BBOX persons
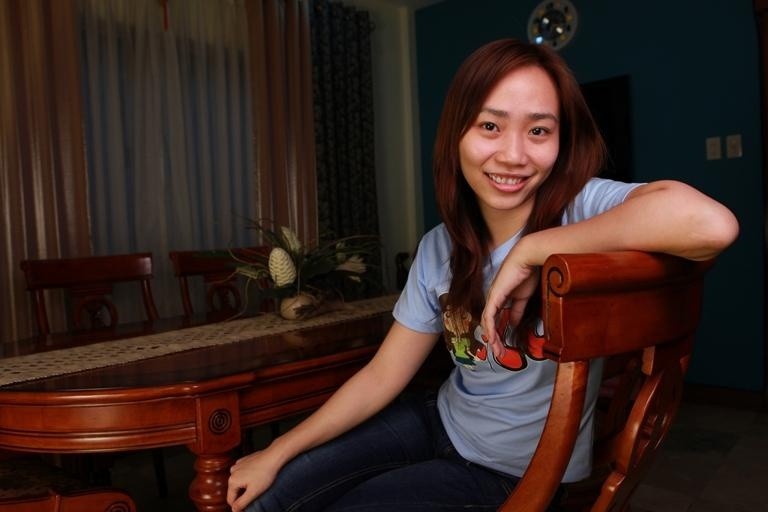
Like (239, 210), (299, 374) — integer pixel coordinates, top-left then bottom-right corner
(226, 39), (741, 511)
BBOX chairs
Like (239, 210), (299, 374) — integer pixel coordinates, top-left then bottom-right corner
(168, 249), (294, 454)
(17, 254), (195, 510)
(499, 250), (706, 511)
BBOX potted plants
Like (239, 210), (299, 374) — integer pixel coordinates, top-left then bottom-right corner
(207, 225), (383, 322)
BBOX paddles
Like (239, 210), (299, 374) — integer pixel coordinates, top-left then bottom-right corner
(628, 426), (741, 512)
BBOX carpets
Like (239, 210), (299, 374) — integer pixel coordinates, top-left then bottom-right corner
(628, 426), (741, 512)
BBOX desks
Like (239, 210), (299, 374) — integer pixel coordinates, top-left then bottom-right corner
(2, 286), (452, 510)
